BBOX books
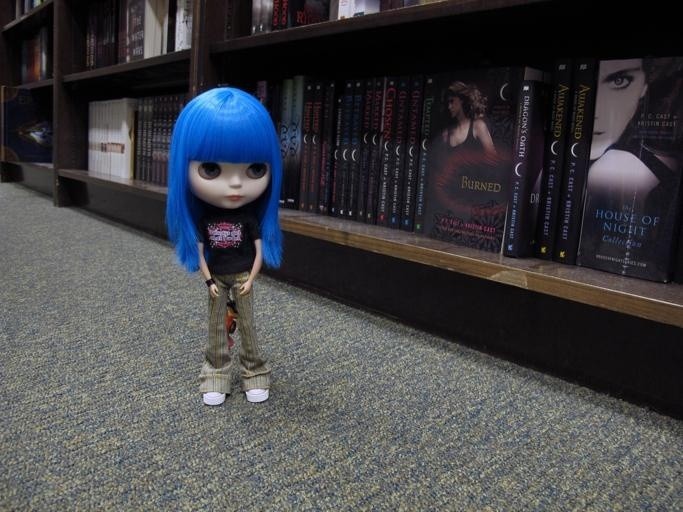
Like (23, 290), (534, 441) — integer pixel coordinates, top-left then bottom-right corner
(3, 1), (680, 284)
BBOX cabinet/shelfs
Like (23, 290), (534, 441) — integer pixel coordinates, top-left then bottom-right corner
(0, 0), (683, 416)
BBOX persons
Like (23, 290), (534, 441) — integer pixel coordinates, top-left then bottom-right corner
(163, 86), (286, 408)
